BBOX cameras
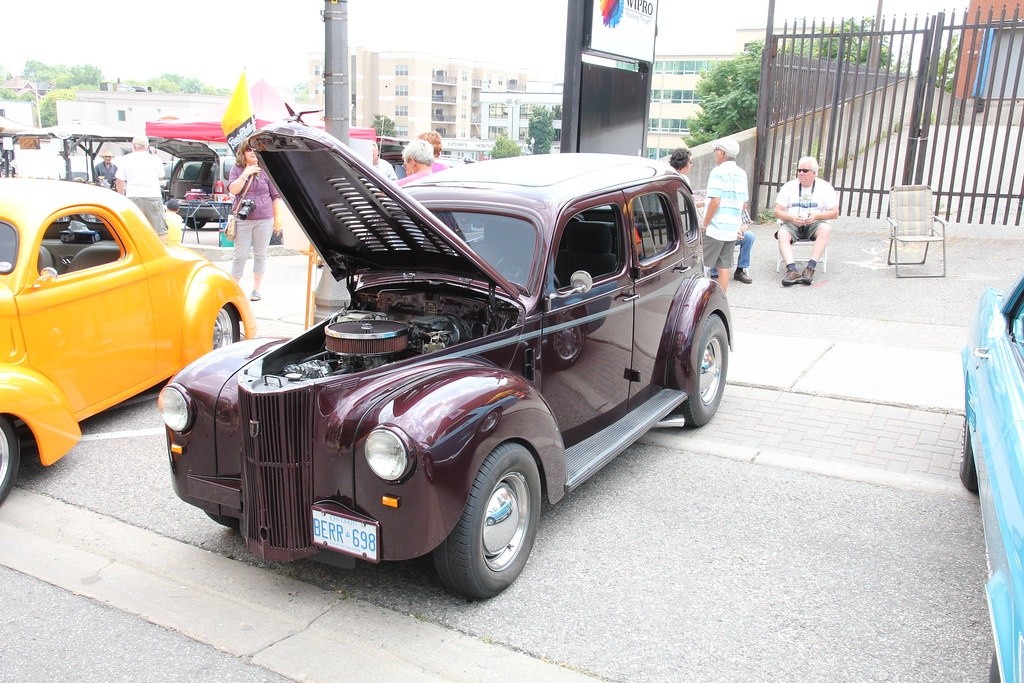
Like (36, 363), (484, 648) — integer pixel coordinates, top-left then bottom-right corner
(798, 224), (809, 234)
(238, 199), (256, 220)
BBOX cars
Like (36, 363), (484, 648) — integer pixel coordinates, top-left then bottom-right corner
(155, 119), (738, 602)
(959, 270), (1024, 683)
(0, 178), (256, 505)
(146, 136), (244, 231)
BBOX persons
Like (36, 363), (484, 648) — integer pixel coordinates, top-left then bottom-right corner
(774, 156), (840, 285)
(226, 138), (281, 301)
(95, 151), (117, 188)
(417, 131), (448, 174)
(695, 137), (749, 293)
(669, 147), (692, 184)
(163, 200), (183, 246)
(391, 140), (433, 187)
(371, 142), (398, 181)
(114, 135), (165, 237)
(710, 202), (755, 284)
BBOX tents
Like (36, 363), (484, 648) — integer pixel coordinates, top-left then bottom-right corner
(146, 116), (377, 143)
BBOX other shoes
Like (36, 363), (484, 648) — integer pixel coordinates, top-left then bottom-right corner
(782, 270), (804, 286)
(801, 266), (815, 285)
(251, 290), (261, 301)
(734, 271), (752, 283)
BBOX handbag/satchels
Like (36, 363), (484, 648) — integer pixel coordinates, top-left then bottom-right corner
(224, 214), (237, 241)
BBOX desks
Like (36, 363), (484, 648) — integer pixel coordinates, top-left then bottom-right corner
(181, 201), (234, 247)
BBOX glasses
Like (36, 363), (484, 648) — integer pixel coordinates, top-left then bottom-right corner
(797, 169), (814, 173)
(246, 147), (253, 152)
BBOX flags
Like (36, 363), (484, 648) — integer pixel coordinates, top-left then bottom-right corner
(220, 72), (255, 158)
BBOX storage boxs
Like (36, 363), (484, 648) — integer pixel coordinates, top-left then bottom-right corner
(220, 232), (234, 247)
(186, 191), (206, 203)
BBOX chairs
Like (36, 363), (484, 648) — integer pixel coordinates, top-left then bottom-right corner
(483, 221), (530, 255)
(0, 242), (53, 275)
(694, 190), (748, 276)
(554, 221), (616, 289)
(887, 185), (947, 278)
(777, 219), (828, 272)
(68, 241), (121, 272)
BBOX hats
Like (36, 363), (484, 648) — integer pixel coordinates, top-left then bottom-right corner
(166, 199), (179, 211)
(712, 138), (740, 156)
(99, 150), (115, 157)
